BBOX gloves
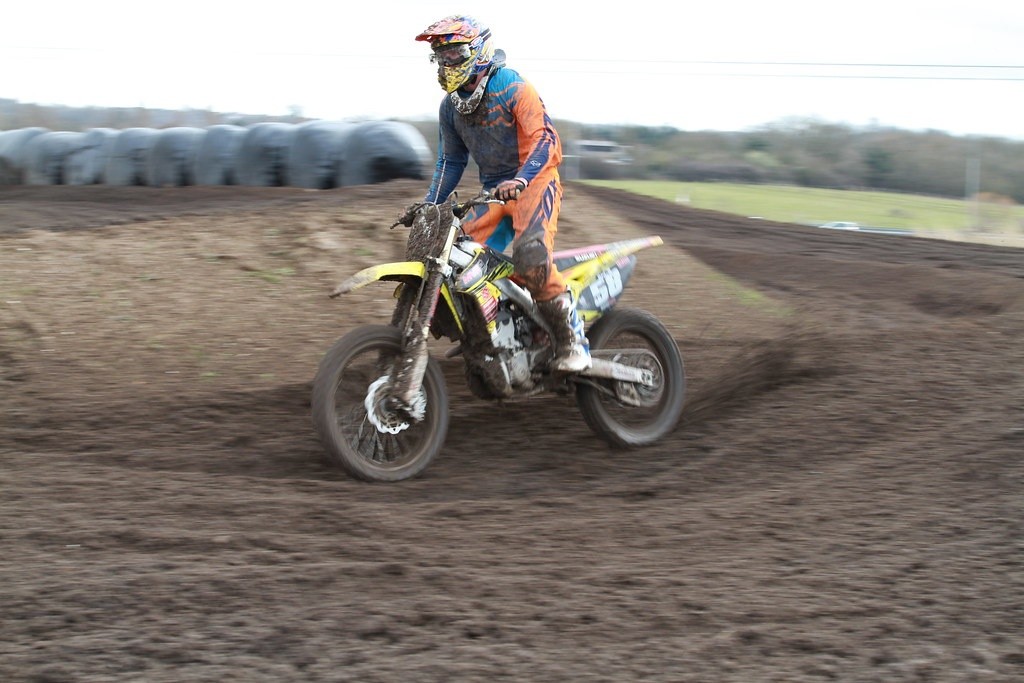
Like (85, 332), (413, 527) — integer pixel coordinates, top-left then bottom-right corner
(493, 177), (529, 202)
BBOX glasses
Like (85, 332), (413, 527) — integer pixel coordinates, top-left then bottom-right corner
(427, 45), (471, 65)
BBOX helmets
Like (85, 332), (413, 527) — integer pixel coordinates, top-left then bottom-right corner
(414, 14), (496, 95)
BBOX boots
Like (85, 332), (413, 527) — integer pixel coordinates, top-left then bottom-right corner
(535, 284), (593, 372)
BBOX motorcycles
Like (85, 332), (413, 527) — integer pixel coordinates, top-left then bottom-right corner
(312, 188), (686, 482)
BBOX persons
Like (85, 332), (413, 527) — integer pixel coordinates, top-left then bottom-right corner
(398, 15), (592, 371)
